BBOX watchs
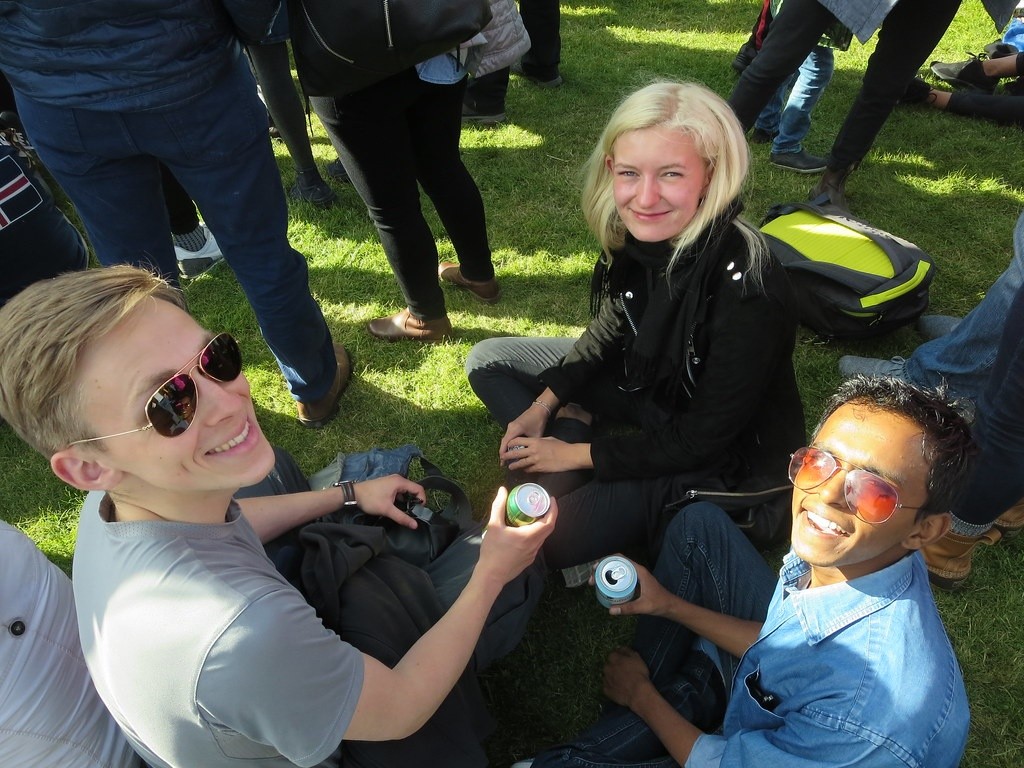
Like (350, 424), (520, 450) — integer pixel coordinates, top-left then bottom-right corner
(334, 480), (357, 508)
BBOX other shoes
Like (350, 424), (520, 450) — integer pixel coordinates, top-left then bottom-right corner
(732, 44), (757, 72)
(285, 178), (335, 207)
(512, 62), (562, 87)
(461, 104), (506, 123)
(330, 159), (349, 181)
(551, 561), (597, 588)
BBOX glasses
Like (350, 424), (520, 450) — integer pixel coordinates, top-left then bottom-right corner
(788, 447), (930, 525)
(68, 332), (243, 448)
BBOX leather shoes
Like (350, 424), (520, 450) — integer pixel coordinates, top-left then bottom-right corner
(769, 144), (828, 172)
(366, 307), (453, 342)
(750, 126), (779, 143)
(437, 259), (502, 304)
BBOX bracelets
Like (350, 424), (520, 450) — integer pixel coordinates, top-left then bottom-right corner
(532, 400), (554, 417)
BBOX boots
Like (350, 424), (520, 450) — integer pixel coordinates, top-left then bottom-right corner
(915, 520), (1002, 591)
(997, 498), (1024, 542)
(810, 156), (858, 213)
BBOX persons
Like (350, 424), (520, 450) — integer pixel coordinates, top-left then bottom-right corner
(837, 205), (1024, 588)
(465, 81), (806, 570)
(726, 0), (1023, 219)
(512, 372), (971, 768)
(0, 519), (141, 768)
(896, 52), (1024, 123)
(0, 0), (353, 426)
(461, 0), (562, 124)
(244, 0), (502, 343)
(0, 264), (558, 768)
(0, 144), (89, 305)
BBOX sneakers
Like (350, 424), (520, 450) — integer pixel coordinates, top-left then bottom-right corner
(930, 52), (1000, 93)
(296, 344), (352, 428)
(838, 354), (908, 384)
(919, 314), (963, 339)
(173, 224), (224, 280)
(899, 75), (937, 105)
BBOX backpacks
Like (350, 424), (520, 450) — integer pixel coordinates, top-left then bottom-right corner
(753, 193), (934, 341)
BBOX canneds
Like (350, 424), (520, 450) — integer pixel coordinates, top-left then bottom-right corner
(481, 482), (550, 539)
(506, 444), (527, 472)
(594, 555), (637, 609)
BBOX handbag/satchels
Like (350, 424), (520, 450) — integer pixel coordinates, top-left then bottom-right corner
(285, 0), (493, 98)
(308, 444), (419, 524)
